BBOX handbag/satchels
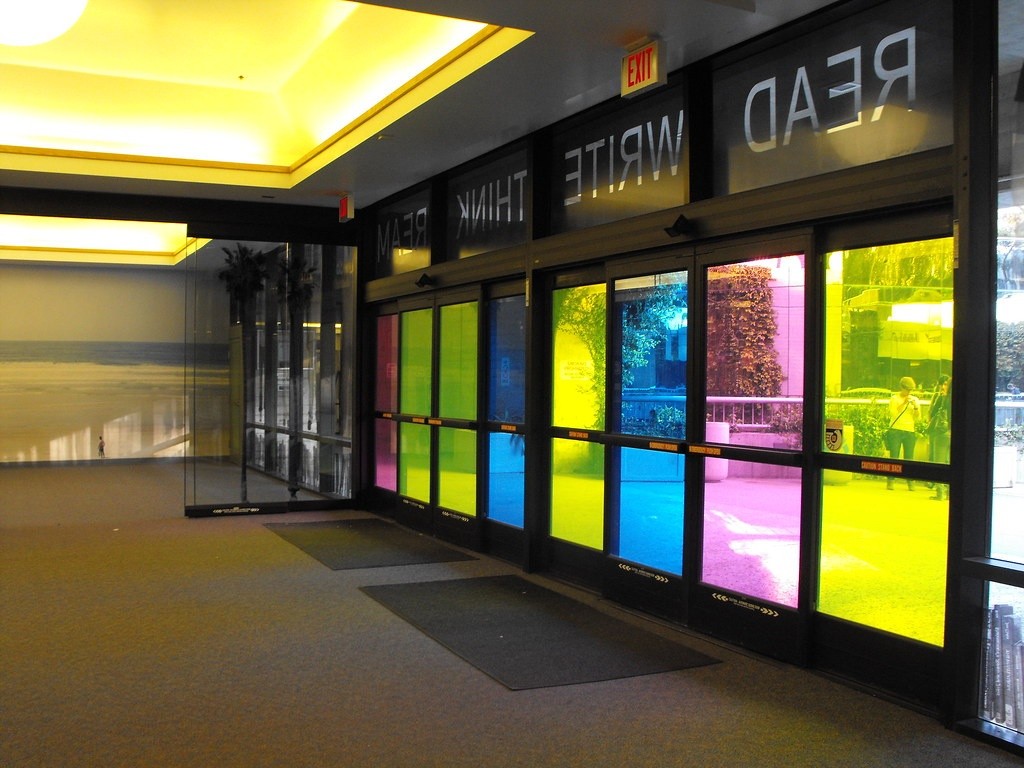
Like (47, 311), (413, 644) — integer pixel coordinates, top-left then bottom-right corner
(883, 432), (890, 450)
(923, 408), (948, 436)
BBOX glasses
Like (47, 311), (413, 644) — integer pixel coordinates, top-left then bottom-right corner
(902, 386), (915, 392)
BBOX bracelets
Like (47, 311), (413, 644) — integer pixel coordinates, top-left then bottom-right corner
(914, 407), (919, 410)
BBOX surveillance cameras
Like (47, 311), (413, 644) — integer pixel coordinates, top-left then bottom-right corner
(415, 273), (430, 287)
(664, 215), (688, 239)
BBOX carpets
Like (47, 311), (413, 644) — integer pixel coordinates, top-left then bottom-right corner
(357, 574), (726, 690)
(263, 518), (480, 572)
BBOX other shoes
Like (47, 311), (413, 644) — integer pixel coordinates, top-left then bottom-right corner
(908, 480), (916, 491)
(887, 479), (894, 490)
(930, 494), (944, 501)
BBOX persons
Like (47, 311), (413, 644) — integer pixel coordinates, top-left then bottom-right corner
(1007, 383), (1020, 399)
(926, 375), (952, 500)
(886, 376), (920, 491)
(98, 436), (105, 458)
